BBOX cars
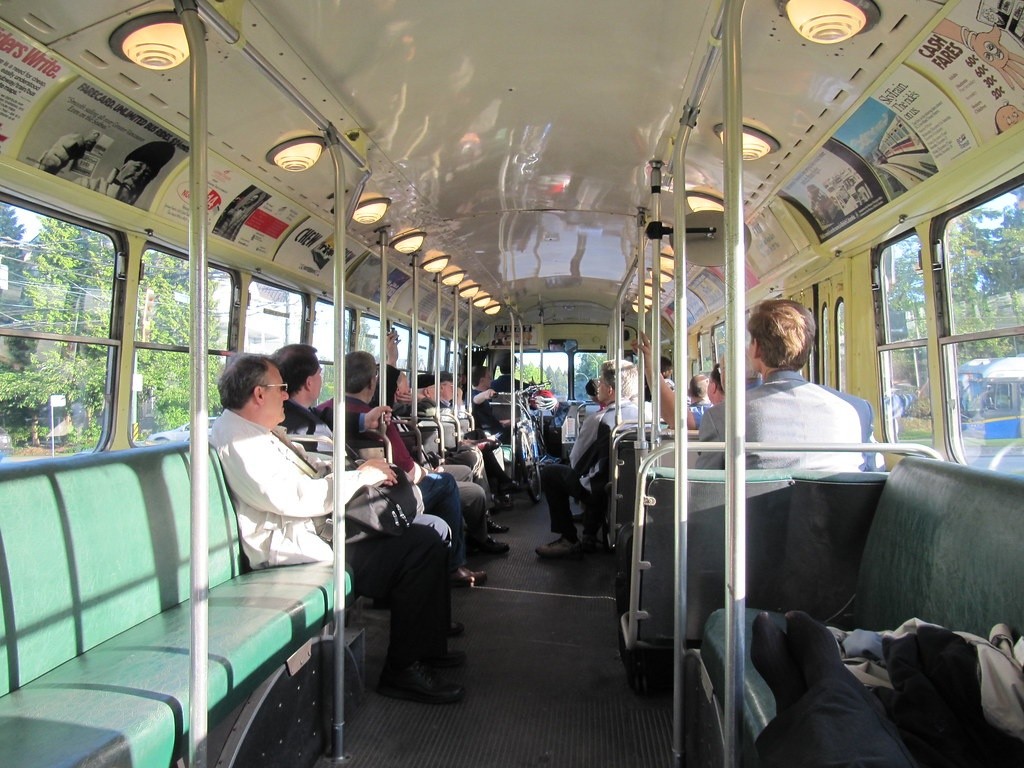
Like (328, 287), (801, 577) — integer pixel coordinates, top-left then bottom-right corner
(147, 416), (218, 445)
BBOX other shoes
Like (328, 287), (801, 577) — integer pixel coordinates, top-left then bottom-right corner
(508, 480), (520, 489)
(499, 494), (513, 511)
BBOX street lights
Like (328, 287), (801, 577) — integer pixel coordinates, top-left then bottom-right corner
(577, 353), (598, 378)
(575, 373), (590, 401)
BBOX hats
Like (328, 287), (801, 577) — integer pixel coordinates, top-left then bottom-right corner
(418, 374), (443, 388)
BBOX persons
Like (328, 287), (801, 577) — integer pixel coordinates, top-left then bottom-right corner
(534, 337), (709, 558)
(491, 354), (530, 392)
(639, 331), (762, 431)
(694, 300), (886, 471)
(272, 344), (465, 639)
(368, 331), (528, 554)
(218, 195), (260, 241)
(84, 132), (100, 152)
(749, 612), (919, 768)
(318, 351), (486, 588)
(209, 355), (469, 702)
(37, 133), (175, 205)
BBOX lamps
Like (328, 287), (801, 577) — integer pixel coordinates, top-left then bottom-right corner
(419, 256), (451, 273)
(482, 304), (502, 315)
(632, 303), (650, 314)
(442, 271), (467, 286)
(686, 191), (725, 213)
(713, 124), (780, 161)
(108, 11), (207, 70)
(460, 284), (480, 297)
(390, 232), (428, 254)
(644, 283), (666, 298)
(352, 198), (392, 225)
(265, 136), (326, 173)
(776, 0), (881, 45)
(646, 268), (673, 282)
(635, 294), (652, 306)
(660, 253), (674, 269)
(473, 295), (493, 307)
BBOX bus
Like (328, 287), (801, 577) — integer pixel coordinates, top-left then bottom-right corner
(957, 356), (1024, 441)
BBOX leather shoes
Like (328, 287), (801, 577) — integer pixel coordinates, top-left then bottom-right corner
(487, 518), (510, 533)
(479, 538), (510, 555)
(448, 566), (486, 588)
(450, 621), (464, 637)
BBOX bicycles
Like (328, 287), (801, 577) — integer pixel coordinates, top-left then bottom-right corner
(491, 382), (551, 505)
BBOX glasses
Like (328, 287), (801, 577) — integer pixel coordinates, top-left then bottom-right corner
(262, 382), (288, 392)
(484, 376), (492, 380)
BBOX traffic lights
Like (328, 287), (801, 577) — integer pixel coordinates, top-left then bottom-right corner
(142, 321), (153, 351)
(142, 289), (162, 325)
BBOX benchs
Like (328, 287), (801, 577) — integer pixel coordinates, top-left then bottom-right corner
(608, 420), (1024, 740)
(0, 407), (518, 768)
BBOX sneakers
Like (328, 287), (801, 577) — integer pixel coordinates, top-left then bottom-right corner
(434, 652), (466, 669)
(535, 537), (580, 558)
(581, 536), (594, 552)
(376, 665), (463, 705)
